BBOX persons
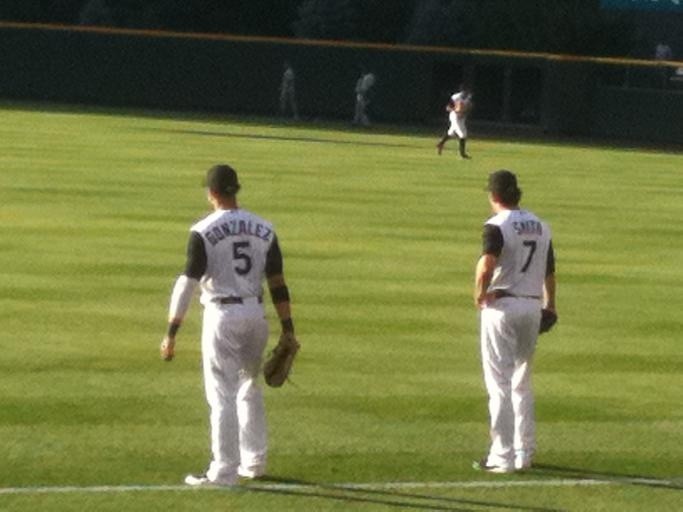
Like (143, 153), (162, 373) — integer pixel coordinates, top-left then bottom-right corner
(437, 81), (474, 158)
(160, 165), (295, 485)
(351, 60), (376, 127)
(279, 60), (301, 122)
(471, 170), (558, 474)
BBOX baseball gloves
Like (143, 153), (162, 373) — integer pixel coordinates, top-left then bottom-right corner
(539, 309), (557, 335)
(263, 331), (300, 388)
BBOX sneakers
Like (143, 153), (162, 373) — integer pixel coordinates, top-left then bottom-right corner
(472, 456), (513, 474)
(236, 463), (266, 478)
(461, 153), (472, 159)
(437, 142), (443, 155)
(515, 453), (532, 471)
(185, 469), (239, 487)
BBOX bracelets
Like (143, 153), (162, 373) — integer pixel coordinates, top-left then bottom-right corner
(165, 322), (180, 337)
(281, 318), (294, 332)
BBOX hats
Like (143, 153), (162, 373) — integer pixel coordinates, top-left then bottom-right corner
(482, 168), (517, 194)
(200, 164), (239, 191)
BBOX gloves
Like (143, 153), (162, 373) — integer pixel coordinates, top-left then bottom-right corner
(159, 333), (174, 361)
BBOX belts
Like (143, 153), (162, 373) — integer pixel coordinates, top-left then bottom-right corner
(494, 290), (539, 299)
(223, 297), (262, 306)
